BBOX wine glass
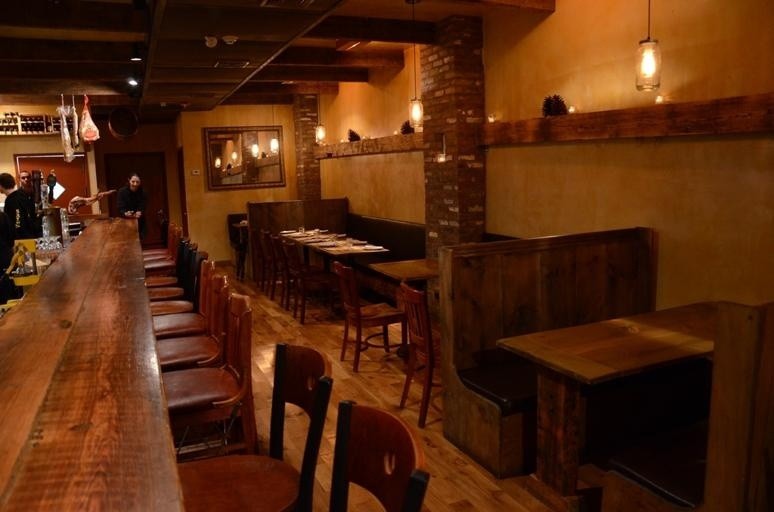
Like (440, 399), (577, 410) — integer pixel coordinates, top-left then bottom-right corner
(34, 236), (64, 251)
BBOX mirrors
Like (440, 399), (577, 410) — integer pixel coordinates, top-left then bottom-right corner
(202, 126), (286, 191)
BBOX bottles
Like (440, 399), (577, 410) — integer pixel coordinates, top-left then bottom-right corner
(0, 112), (77, 131)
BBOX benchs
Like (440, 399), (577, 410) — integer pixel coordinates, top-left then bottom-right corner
(328, 212), (519, 306)
(227, 197), (350, 282)
(438, 225), (657, 480)
(601, 300), (774, 512)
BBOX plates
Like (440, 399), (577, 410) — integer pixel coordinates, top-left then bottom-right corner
(280, 227), (384, 252)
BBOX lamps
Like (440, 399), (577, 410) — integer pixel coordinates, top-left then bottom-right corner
(409, 43), (424, 128)
(636, 0), (662, 91)
(213, 130), (279, 168)
(315, 92), (326, 144)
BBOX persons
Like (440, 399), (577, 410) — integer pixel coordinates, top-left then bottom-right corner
(117, 171), (148, 240)
(18, 171), (34, 199)
(0, 172), (35, 239)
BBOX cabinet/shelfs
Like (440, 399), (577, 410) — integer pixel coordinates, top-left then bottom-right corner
(0, 113), (79, 136)
